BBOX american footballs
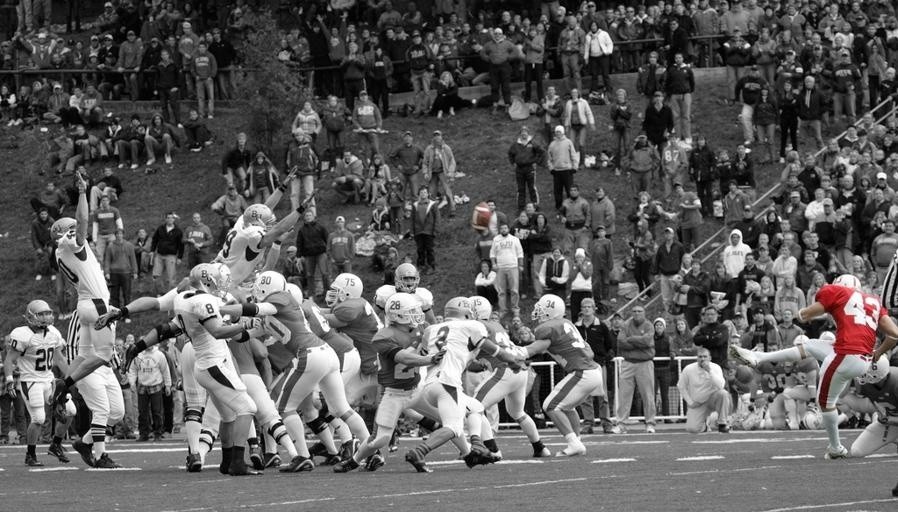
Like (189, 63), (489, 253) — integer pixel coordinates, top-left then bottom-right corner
(472, 200), (491, 234)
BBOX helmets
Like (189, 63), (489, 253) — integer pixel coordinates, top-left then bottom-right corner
(468, 296), (492, 321)
(249, 271), (287, 303)
(190, 263), (232, 294)
(243, 204), (276, 237)
(803, 406), (822, 431)
(25, 299), (55, 330)
(51, 218), (77, 241)
(385, 292), (425, 324)
(332, 273), (362, 300)
(793, 334), (809, 346)
(819, 331), (836, 340)
(531, 294), (564, 322)
(394, 264), (420, 293)
(443, 296), (477, 321)
(287, 283), (303, 306)
(832, 274), (861, 289)
(856, 350), (890, 386)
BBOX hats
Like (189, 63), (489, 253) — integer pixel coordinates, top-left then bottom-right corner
(754, 307), (764, 314)
(56, 30), (134, 43)
(733, 311), (743, 317)
(653, 317), (667, 329)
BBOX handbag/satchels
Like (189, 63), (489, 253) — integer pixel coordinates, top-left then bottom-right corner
(672, 290), (688, 306)
(668, 303), (683, 315)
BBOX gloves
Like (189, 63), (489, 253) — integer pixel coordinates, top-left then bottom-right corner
(6, 376), (16, 398)
(430, 351), (446, 363)
(76, 171), (87, 193)
(279, 165), (297, 193)
(296, 191), (319, 215)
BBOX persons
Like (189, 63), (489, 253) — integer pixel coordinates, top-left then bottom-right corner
(0, 0), (897, 474)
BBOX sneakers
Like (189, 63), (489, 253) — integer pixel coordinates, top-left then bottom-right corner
(47, 378), (69, 424)
(718, 424), (729, 432)
(824, 446), (848, 460)
(93, 308), (123, 330)
(1, 427), (180, 468)
(729, 344), (759, 368)
(7, 115), (215, 176)
(186, 438), (502, 475)
(581, 421), (656, 434)
(121, 344), (138, 374)
(532, 447), (551, 458)
(556, 447), (585, 456)
